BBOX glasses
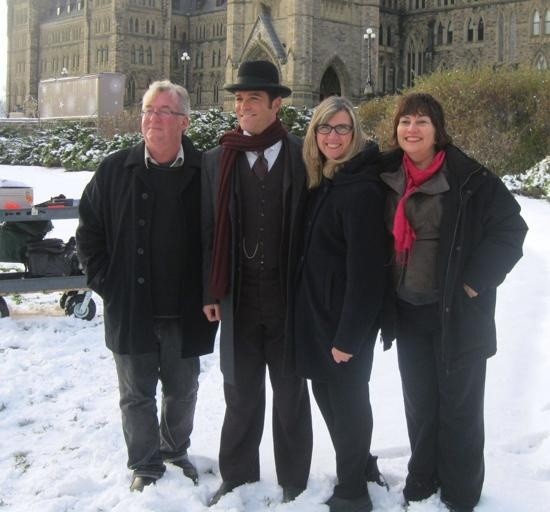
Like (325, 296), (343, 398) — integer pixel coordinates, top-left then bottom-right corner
(140, 108), (185, 120)
(316, 123), (354, 135)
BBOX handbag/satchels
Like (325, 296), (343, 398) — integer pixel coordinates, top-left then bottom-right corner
(19, 235), (85, 277)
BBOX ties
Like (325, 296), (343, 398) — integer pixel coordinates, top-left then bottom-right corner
(250, 148), (269, 182)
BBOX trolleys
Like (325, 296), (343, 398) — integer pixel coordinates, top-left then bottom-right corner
(0, 198), (98, 320)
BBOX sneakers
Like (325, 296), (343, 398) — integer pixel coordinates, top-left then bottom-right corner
(173, 460), (198, 485)
(207, 481), (244, 505)
(281, 486), (307, 504)
(325, 495), (375, 511)
(130, 476), (155, 492)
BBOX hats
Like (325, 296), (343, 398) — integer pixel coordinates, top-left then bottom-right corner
(223, 61), (293, 99)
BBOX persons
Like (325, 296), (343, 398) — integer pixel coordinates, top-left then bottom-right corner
(200, 59), (315, 508)
(294, 96), (388, 511)
(76, 80), (218, 491)
(379, 93), (528, 512)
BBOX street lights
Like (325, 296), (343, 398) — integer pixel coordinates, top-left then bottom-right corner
(60, 67), (69, 77)
(181, 51), (191, 87)
(363, 27), (375, 100)
